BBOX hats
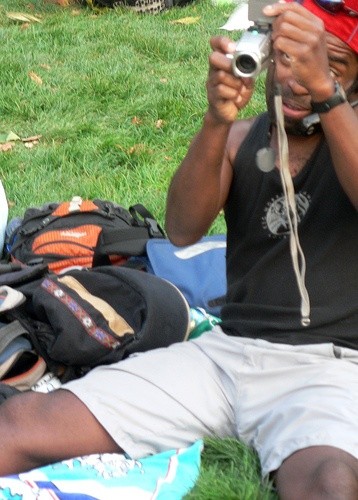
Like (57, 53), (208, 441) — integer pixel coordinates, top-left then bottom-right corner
(284, 0), (358, 54)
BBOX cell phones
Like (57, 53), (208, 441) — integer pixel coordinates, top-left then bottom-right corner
(300, 99), (358, 137)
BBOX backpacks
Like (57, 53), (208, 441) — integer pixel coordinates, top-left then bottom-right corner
(0, 311), (46, 404)
(4, 195), (166, 275)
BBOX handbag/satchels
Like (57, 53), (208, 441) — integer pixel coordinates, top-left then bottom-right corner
(145, 233), (228, 317)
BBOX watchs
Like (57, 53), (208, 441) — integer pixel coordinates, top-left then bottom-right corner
(310, 80), (348, 113)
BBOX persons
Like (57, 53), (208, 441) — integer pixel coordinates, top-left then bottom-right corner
(1, 0), (358, 496)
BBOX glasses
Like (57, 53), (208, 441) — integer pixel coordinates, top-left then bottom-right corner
(313, 0), (358, 20)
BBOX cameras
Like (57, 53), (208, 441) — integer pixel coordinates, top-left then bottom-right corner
(223, 0), (280, 77)
